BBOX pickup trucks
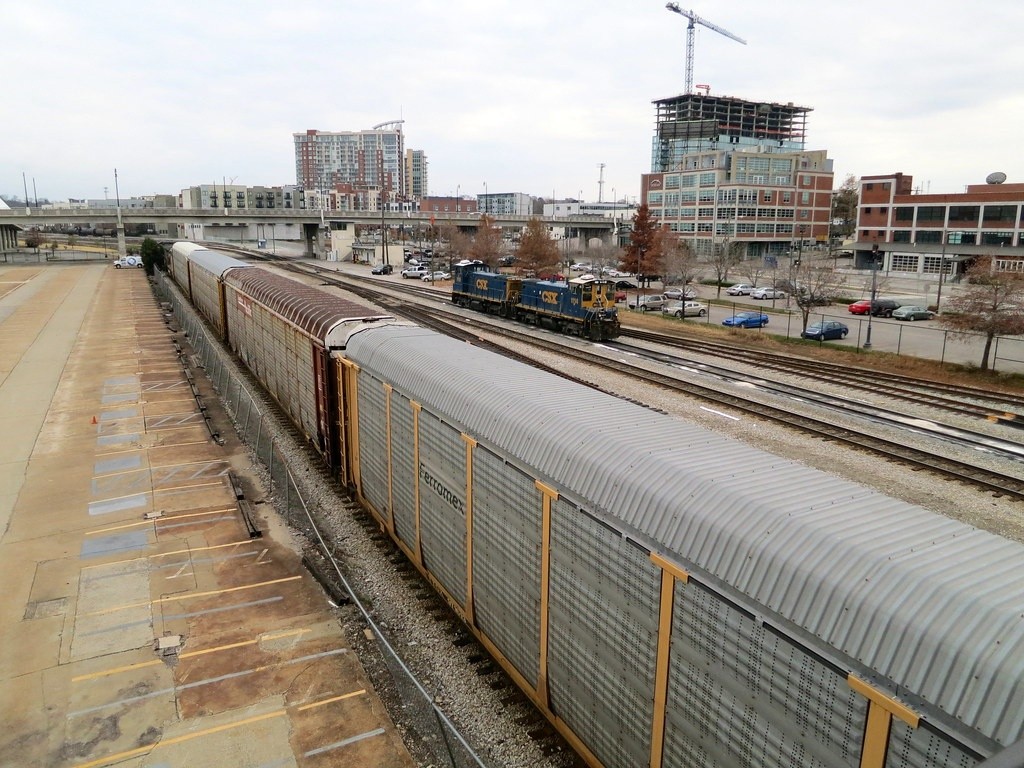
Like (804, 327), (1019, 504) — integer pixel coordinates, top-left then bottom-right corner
(402, 266), (430, 279)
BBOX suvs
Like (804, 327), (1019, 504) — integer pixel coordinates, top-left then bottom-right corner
(629, 295), (669, 311)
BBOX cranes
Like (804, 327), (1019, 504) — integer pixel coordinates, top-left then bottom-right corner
(665, 2), (747, 96)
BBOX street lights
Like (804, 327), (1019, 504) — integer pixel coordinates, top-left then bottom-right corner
(455, 177), (642, 220)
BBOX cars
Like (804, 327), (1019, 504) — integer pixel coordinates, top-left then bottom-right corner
(664, 286), (696, 301)
(750, 287), (786, 300)
(372, 264), (393, 275)
(892, 306), (934, 322)
(113, 257), (144, 269)
(422, 271), (451, 282)
(849, 300), (871, 316)
(403, 245), (483, 270)
(801, 320), (849, 342)
(615, 280), (638, 303)
(662, 300), (708, 319)
(871, 298), (902, 318)
(495, 254), (662, 282)
(726, 283), (754, 296)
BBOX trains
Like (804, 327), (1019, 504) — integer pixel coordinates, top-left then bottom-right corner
(167, 239), (1024, 768)
(450, 259), (621, 343)
